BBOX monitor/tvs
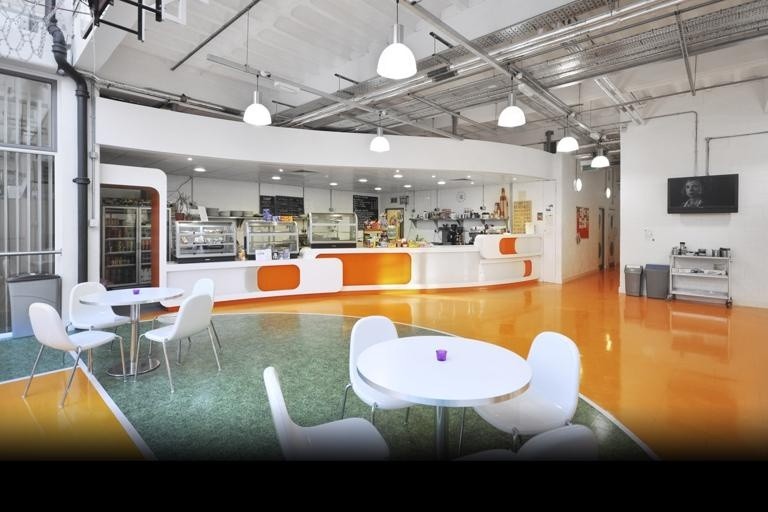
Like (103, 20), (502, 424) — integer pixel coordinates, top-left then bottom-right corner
(667, 174), (738, 214)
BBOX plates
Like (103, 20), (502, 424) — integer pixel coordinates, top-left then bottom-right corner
(207, 208), (254, 217)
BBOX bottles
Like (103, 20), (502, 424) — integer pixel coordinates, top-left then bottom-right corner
(106, 219), (150, 265)
(273, 248), (290, 260)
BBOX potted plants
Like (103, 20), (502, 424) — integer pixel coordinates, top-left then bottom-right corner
(175, 189), (193, 221)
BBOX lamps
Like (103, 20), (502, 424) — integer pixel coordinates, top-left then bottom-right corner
(370, 114), (390, 154)
(376, 0), (417, 81)
(497, 76), (527, 129)
(243, 76), (271, 126)
(556, 116), (579, 152)
(590, 142), (610, 168)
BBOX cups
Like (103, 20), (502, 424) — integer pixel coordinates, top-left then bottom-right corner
(672, 242), (731, 257)
(436, 349), (447, 362)
(131, 287), (140, 295)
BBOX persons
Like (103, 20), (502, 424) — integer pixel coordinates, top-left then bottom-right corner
(681, 178), (708, 209)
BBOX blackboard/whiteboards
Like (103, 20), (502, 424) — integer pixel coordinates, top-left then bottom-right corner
(353, 195), (378, 230)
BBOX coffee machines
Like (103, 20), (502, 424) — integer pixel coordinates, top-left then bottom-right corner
(448, 224), (464, 245)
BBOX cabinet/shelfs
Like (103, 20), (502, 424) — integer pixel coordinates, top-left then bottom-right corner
(172, 213), (358, 263)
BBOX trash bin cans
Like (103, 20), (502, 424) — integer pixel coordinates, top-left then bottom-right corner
(624, 265), (643, 297)
(6, 275), (61, 339)
(645, 264), (670, 299)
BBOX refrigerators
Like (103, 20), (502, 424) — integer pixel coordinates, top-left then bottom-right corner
(100, 206), (153, 287)
